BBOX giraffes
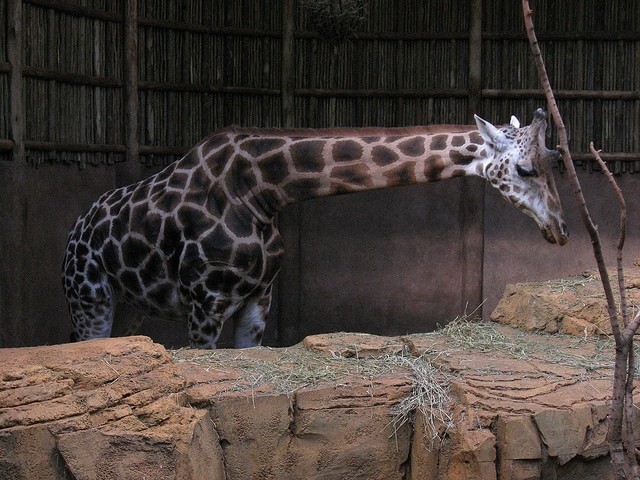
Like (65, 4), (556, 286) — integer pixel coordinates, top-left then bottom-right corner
(59, 104), (573, 351)
